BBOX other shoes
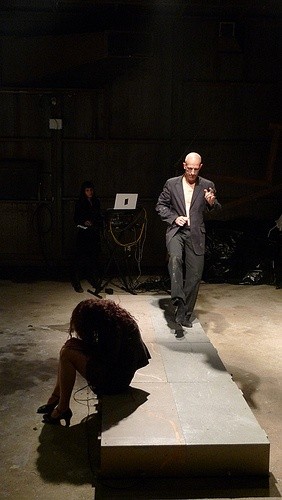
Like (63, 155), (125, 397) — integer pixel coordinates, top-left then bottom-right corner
(172, 297), (184, 307)
(182, 320), (193, 328)
(71, 280), (84, 292)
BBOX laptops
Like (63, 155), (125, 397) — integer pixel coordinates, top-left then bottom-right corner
(106, 193), (138, 210)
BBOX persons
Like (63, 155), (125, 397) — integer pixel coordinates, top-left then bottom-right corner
(37, 298), (150, 427)
(155, 152), (221, 327)
(68, 180), (104, 292)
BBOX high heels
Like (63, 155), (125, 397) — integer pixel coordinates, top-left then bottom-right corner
(37, 394), (59, 414)
(43, 408), (72, 425)
